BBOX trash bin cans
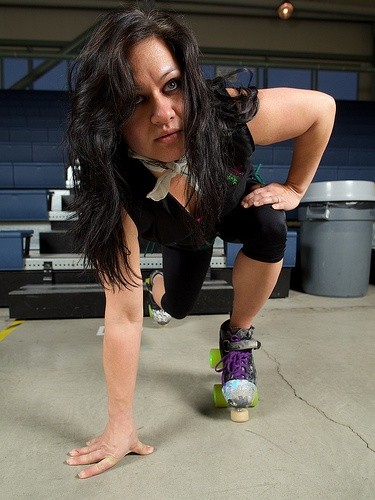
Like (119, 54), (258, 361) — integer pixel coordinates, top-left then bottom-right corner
(296, 179), (375, 297)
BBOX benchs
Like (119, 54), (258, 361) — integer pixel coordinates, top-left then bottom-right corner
(0, 86), (375, 271)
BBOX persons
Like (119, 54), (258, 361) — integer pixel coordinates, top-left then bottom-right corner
(64, 0), (336, 479)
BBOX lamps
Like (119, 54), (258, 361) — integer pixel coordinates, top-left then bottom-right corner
(276, 0), (296, 21)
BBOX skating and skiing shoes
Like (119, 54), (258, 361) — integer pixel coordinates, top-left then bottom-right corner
(145, 270), (171, 325)
(210, 318), (261, 422)
